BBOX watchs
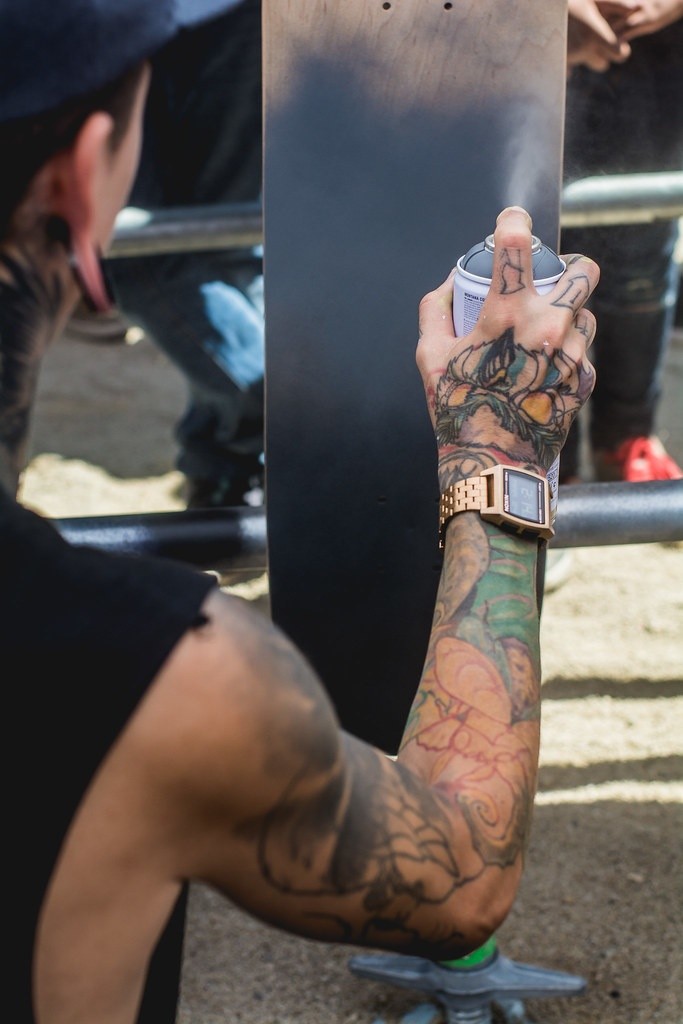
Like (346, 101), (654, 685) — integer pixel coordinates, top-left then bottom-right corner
(438, 463), (555, 551)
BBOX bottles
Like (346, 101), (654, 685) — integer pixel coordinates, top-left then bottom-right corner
(453, 231), (567, 524)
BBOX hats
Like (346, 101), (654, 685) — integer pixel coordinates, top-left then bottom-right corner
(0, 0), (240, 121)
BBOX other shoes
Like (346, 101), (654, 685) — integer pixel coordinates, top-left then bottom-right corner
(597, 436), (683, 484)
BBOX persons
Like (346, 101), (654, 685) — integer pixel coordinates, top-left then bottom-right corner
(0, 0), (602, 1023)
(64, 1), (683, 593)
